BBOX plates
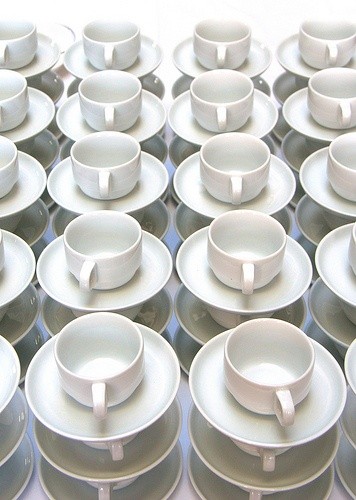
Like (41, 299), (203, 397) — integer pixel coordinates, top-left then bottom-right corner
(0, 32), (356, 500)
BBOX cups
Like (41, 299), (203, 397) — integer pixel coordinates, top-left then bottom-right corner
(0, 134), (19, 200)
(223, 316), (316, 426)
(231, 437), (292, 471)
(201, 133), (271, 205)
(0, 69), (28, 133)
(81, 19), (140, 70)
(54, 312), (144, 419)
(84, 433), (138, 462)
(192, 18), (251, 70)
(0, 17), (38, 69)
(83, 477), (136, 499)
(63, 210), (142, 291)
(191, 69), (254, 132)
(308, 66), (355, 129)
(299, 19), (355, 69)
(238, 485), (276, 500)
(328, 131), (355, 203)
(78, 70), (142, 132)
(207, 210), (287, 293)
(70, 131), (141, 200)
